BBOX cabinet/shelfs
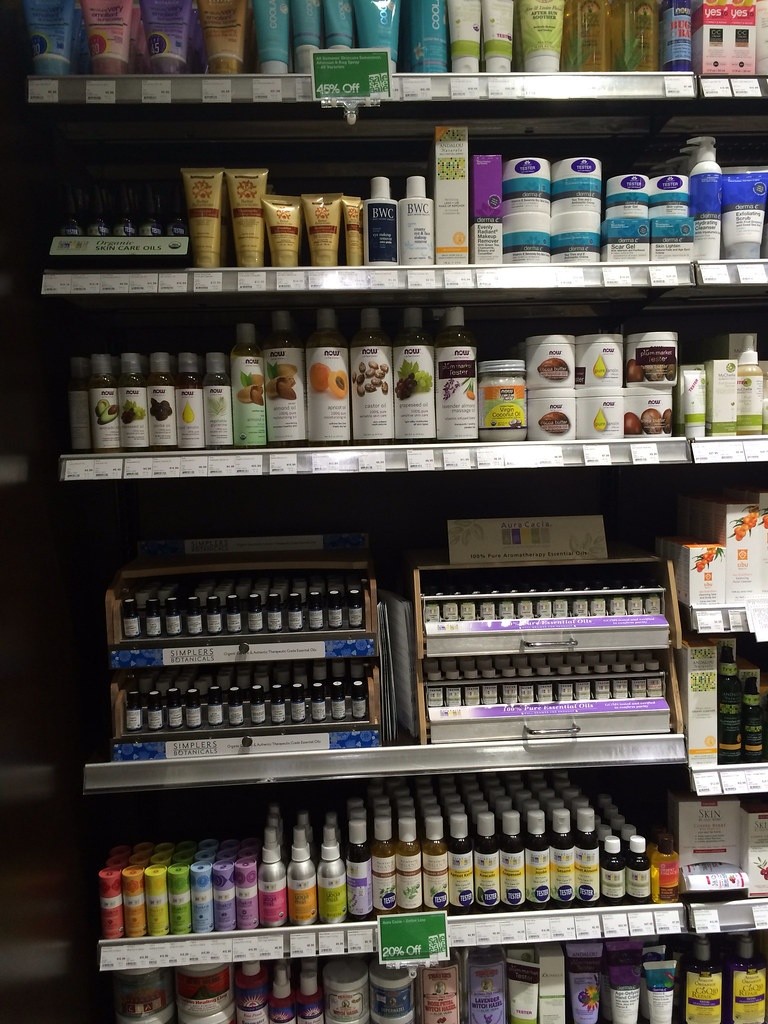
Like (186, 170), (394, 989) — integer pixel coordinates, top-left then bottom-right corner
(641, 75), (768, 934)
(405, 541), (684, 745)
(105, 533), (382, 764)
(26, 71), (697, 972)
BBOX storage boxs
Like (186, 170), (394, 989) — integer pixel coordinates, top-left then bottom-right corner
(601, 218), (696, 266)
(469, 155), (504, 264)
(427, 125), (467, 265)
(656, 480), (768, 609)
(675, 634), (760, 768)
(507, 942), (566, 1023)
(690, 0), (756, 75)
(674, 365), (704, 436)
(703, 360), (737, 437)
(713, 333), (758, 364)
(667, 788), (768, 899)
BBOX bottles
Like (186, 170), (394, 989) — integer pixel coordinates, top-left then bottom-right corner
(399, 176), (435, 264)
(422, 586), (663, 708)
(363, 176), (399, 266)
(123, 576), (368, 733)
(98, 771), (679, 1024)
(736, 335), (768, 435)
(51, 180), (187, 269)
(71, 306), (478, 453)
